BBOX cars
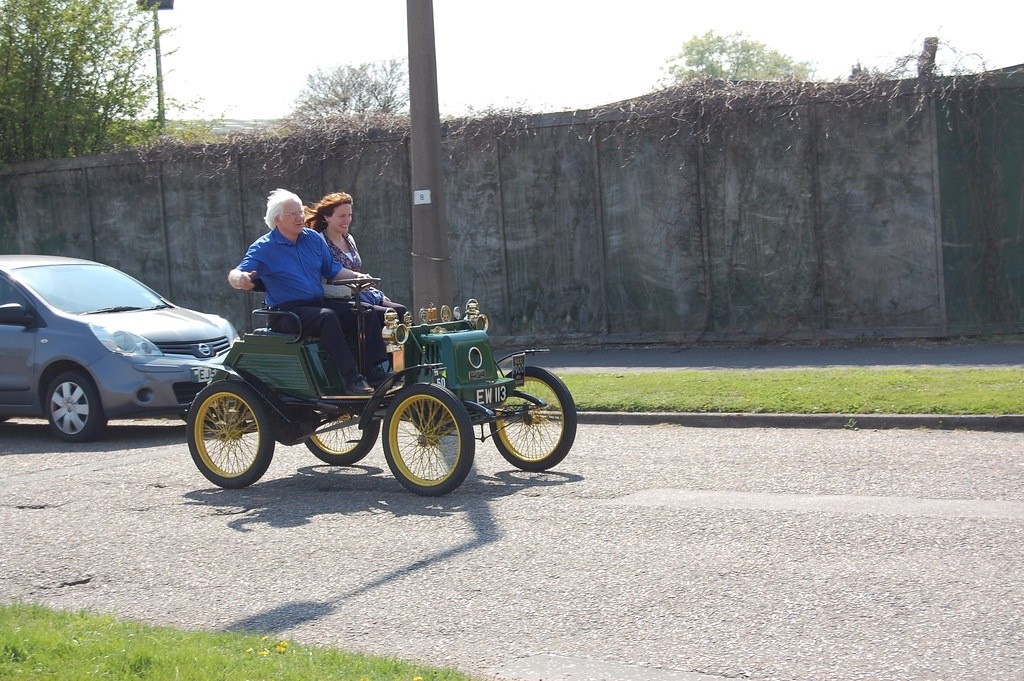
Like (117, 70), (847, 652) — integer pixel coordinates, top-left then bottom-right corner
(0, 253), (242, 444)
(184, 276), (579, 497)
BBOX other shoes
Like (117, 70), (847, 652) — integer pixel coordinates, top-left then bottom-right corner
(367, 371), (394, 387)
(345, 378), (375, 396)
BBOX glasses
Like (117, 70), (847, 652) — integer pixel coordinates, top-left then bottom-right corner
(283, 210), (308, 218)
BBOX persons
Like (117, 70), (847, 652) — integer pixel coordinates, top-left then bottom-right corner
(302, 191), (416, 384)
(227, 188), (394, 395)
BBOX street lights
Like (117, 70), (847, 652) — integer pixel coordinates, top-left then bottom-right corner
(135, 0), (175, 126)
